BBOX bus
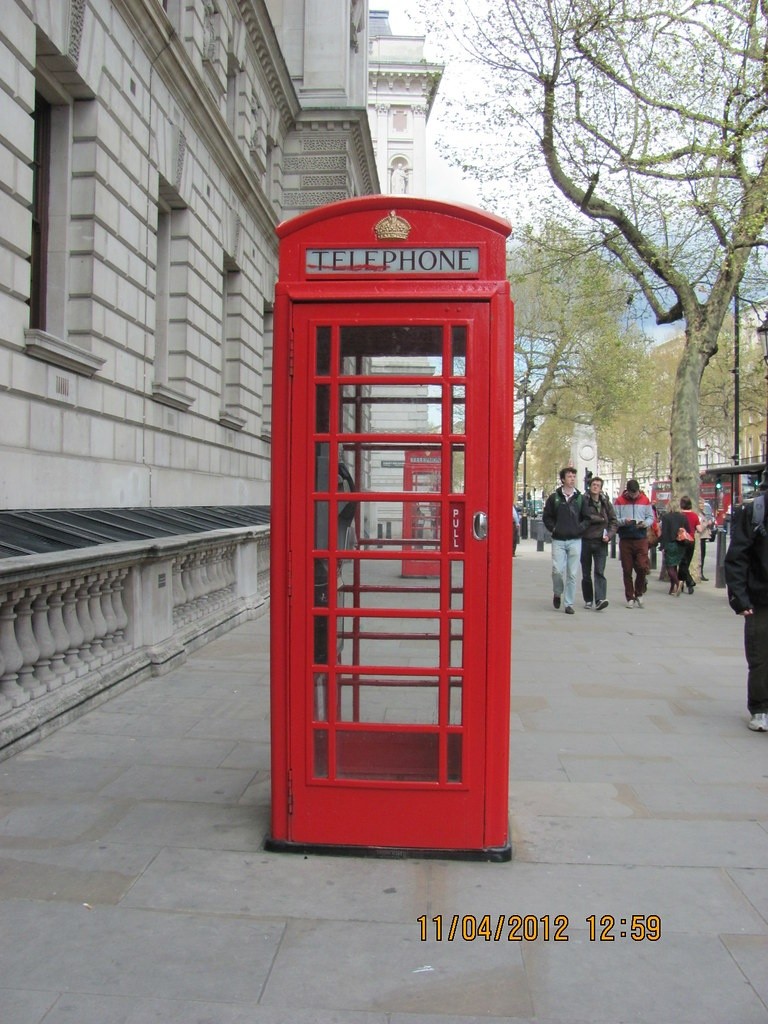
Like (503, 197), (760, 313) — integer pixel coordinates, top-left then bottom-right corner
(650, 472), (760, 540)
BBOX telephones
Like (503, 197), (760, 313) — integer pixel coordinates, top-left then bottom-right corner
(315, 442), (356, 559)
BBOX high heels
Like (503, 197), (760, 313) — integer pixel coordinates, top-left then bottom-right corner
(688, 581), (696, 595)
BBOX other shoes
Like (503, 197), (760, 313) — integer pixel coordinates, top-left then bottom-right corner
(668, 589), (676, 595)
(553, 595), (561, 609)
(565, 606), (575, 614)
(675, 581), (683, 596)
(701, 574), (709, 581)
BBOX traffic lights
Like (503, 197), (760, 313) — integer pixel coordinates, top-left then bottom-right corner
(585, 470), (593, 493)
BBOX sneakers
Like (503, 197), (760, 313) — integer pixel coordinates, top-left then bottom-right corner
(748, 713), (768, 731)
(595, 599), (609, 610)
(584, 601), (593, 609)
(626, 600), (635, 609)
(635, 596), (643, 608)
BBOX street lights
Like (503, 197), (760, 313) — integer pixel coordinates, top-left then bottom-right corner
(704, 444), (710, 469)
(654, 452), (660, 481)
(520, 371), (531, 539)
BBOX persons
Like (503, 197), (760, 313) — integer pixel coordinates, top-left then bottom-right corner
(723, 484), (768, 732)
(513, 467), (716, 614)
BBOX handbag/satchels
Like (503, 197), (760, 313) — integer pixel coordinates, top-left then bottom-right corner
(676, 514), (694, 545)
